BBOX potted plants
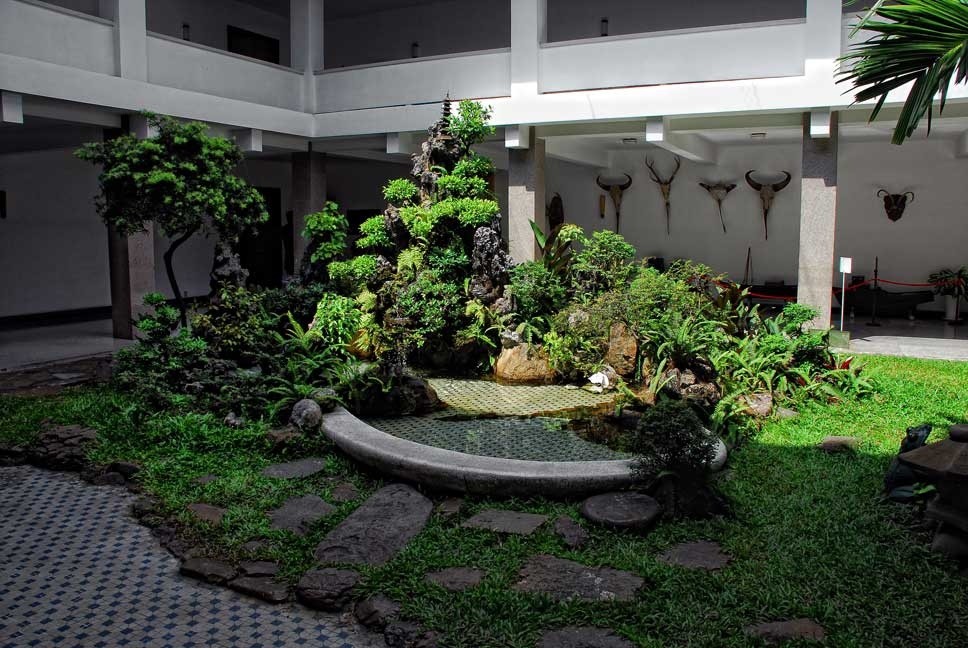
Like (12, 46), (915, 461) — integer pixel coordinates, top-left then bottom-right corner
(928, 265), (968, 321)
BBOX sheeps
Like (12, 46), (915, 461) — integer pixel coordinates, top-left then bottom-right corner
(596, 152), (792, 241)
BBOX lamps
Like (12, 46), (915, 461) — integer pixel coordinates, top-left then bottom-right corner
(412, 43), (419, 58)
(183, 24), (190, 40)
(601, 19), (608, 36)
